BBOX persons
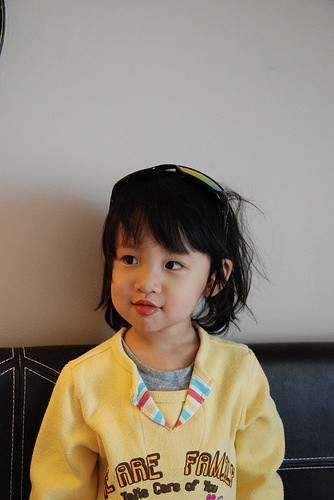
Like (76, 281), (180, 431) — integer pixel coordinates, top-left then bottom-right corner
(26, 164), (288, 500)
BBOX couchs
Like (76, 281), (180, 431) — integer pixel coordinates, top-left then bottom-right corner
(0, 340), (334, 500)
